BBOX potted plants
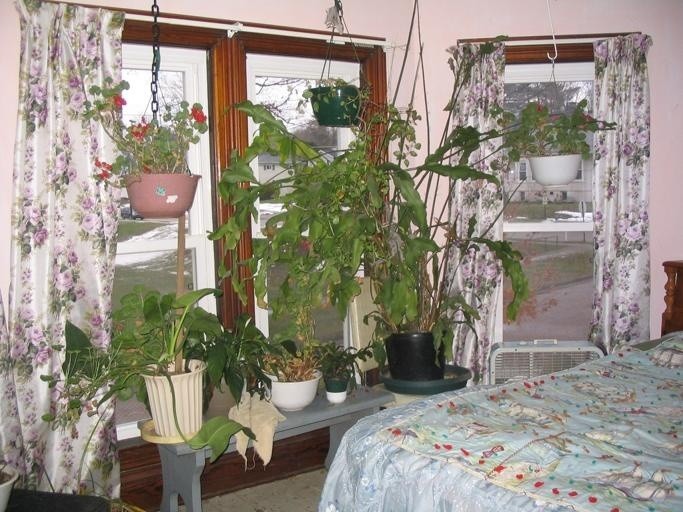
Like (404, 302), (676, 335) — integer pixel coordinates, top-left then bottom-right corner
(260, 347), (325, 412)
(209, 99), (527, 382)
(498, 105), (617, 185)
(144, 312), (284, 414)
(323, 98), (619, 409)
(41, 284), (258, 463)
(308, 341), (376, 405)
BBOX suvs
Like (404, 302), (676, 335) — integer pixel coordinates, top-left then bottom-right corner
(121, 202), (145, 221)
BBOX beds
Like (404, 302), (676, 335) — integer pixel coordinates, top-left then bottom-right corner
(314, 259), (683, 512)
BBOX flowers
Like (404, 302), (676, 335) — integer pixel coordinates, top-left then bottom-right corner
(82, 75), (209, 188)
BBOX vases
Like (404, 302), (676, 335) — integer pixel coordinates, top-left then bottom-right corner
(120, 174), (203, 219)
(307, 84), (368, 127)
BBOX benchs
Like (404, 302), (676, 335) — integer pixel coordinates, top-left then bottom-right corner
(138, 382), (397, 512)
